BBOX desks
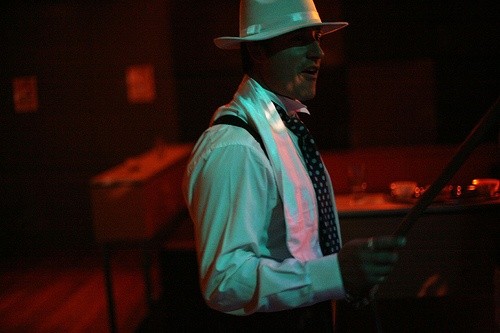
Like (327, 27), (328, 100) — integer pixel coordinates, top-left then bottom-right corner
(90, 145), (195, 333)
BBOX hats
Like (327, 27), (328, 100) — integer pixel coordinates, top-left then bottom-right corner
(214, 0), (348, 49)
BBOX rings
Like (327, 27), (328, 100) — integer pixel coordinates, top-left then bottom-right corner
(366, 238), (374, 251)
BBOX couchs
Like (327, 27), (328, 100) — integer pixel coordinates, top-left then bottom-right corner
(322, 142), (500, 218)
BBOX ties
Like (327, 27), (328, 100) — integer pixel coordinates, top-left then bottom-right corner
(286, 116), (341, 257)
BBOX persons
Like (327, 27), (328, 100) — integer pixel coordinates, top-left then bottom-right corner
(183, 0), (407, 333)
